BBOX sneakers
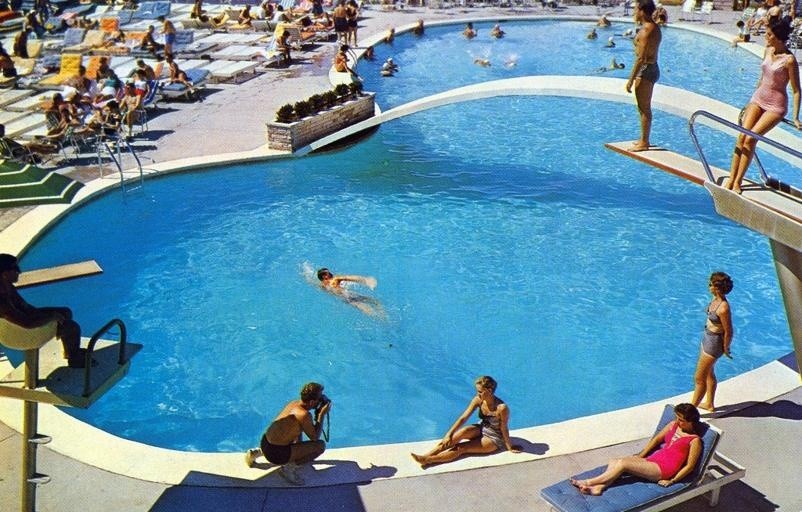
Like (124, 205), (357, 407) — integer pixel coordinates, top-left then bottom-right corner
(279, 465), (305, 485)
(246, 448), (261, 466)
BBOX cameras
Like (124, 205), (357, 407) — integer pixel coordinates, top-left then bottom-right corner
(316, 396), (330, 412)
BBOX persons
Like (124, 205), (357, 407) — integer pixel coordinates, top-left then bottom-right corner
(244, 382), (333, 486)
(589, 16), (634, 70)
(569, 403), (703, 496)
(365, 18), (504, 75)
(627, 0), (662, 152)
(723, 18), (802, 194)
(317, 268), (384, 318)
(732, 0), (782, 47)
(652, 3), (667, 25)
(411, 376), (523, 466)
(0, 0), (360, 159)
(691, 271), (734, 412)
(0, 254), (98, 368)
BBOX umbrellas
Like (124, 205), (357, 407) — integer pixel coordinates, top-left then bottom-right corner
(0, 158), (84, 207)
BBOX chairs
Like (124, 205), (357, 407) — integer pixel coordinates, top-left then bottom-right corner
(741, 6), (802, 66)
(678, 0), (714, 24)
(0, 318), (61, 388)
(0, 0), (362, 167)
(541, 405), (745, 512)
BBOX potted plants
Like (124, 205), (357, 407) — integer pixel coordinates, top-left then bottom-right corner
(266, 80), (375, 153)
